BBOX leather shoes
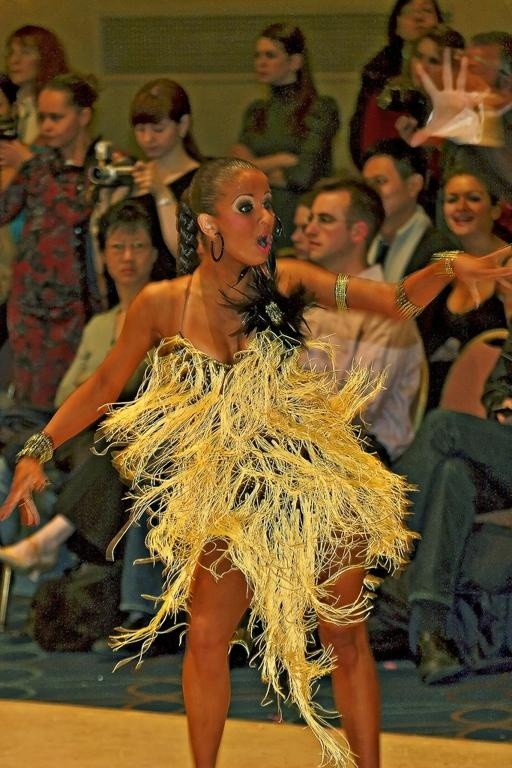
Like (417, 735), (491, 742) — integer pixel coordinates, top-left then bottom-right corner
(87, 613), (180, 659)
(411, 623), (469, 688)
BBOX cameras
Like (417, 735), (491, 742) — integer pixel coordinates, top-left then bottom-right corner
(88, 141), (135, 186)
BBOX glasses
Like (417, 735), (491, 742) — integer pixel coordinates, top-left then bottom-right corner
(306, 213), (358, 227)
(107, 239), (154, 254)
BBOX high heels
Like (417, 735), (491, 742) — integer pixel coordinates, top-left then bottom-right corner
(1, 535), (62, 588)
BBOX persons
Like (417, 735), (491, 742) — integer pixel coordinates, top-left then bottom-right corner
(408, 46), (511, 158)
(409, 31), (512, 200)
(289, 193), (323, 264)
(0, 77), (16, 141)
(361, 329), (511, 683)
(0, 199), (161, 582)
(296, 179), (428, 470)
(358, 24), (467, 225)
(348, 0), (445, 171)
(231, 24), (341, 256)
(82, 79), (205, 311)
(416, 170), (511, 410)
(0, 25), (69, 184)
(357, 140), (459, 284)
(0, 155), (511, 768)
(0, 72), (137, 409)
